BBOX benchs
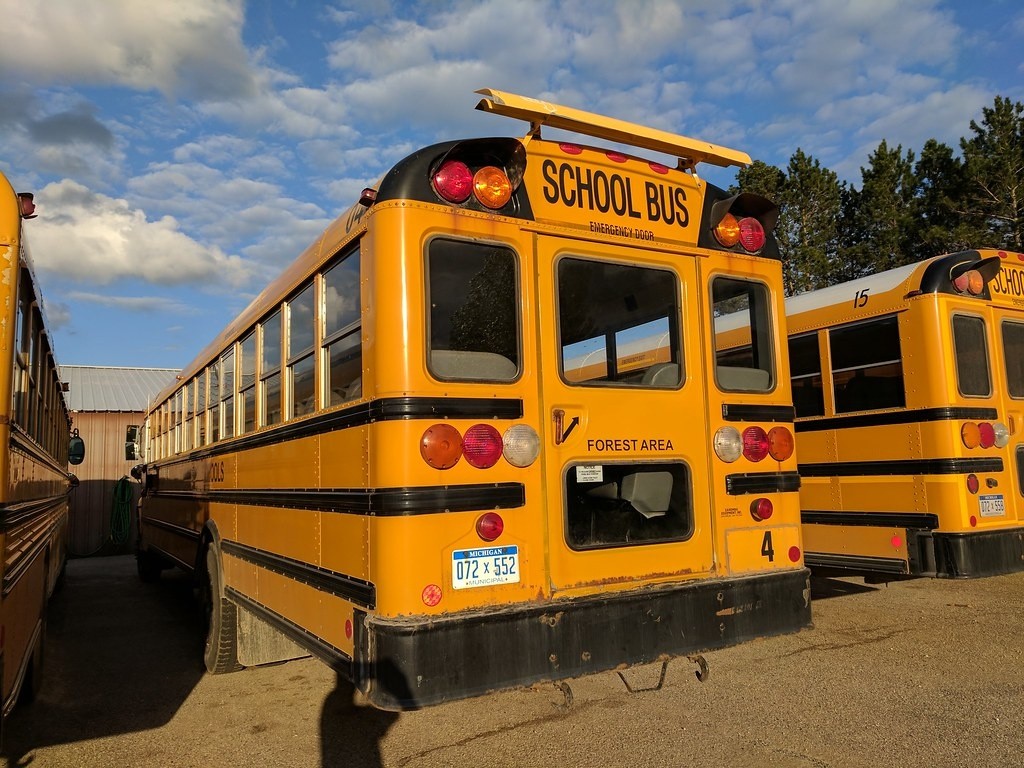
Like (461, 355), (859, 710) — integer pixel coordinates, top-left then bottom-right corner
(621, 363), (768, 511)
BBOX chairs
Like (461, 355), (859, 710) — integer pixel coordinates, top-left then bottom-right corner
(344, 377), (362, 402)
(305, 387), (346, 415)
(431, 349), (516, 379)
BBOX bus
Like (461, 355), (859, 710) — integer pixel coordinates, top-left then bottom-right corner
(117, 83), (811, 713)
(2, 174), (85, 768)
(565, 248), (1024, 588)
